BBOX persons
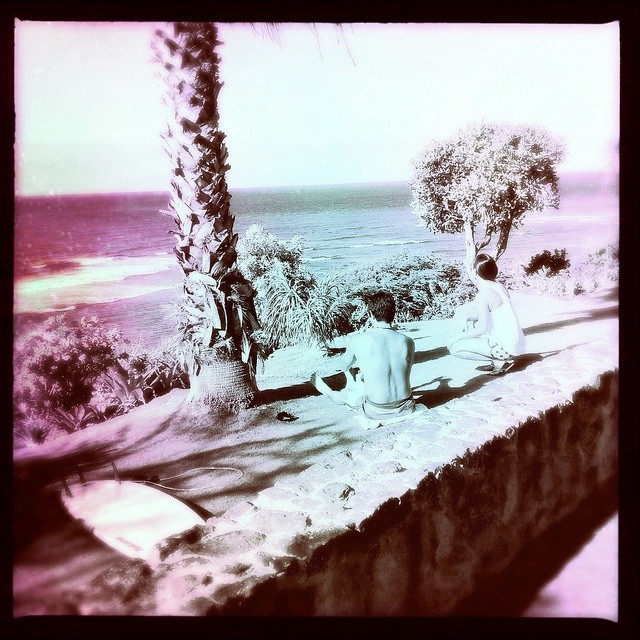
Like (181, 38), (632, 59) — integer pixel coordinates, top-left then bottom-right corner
(448, 253), (524, 376)
(308, 291), (415, 422)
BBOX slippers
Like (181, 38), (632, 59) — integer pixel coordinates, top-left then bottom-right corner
(490, 362), (514, 376)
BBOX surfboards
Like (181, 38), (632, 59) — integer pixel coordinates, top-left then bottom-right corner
(55, 470), (206, 569)
